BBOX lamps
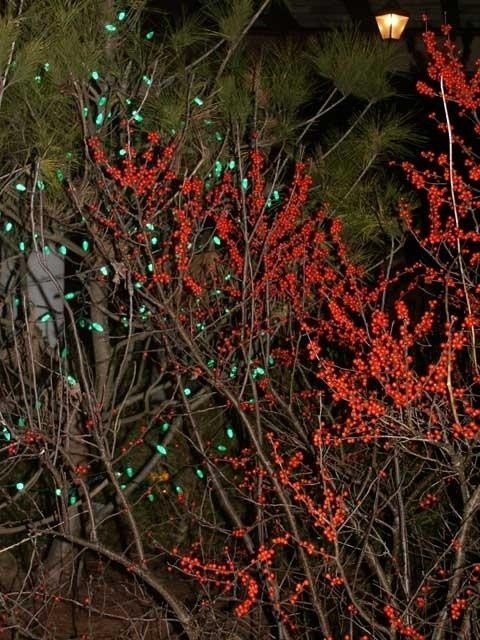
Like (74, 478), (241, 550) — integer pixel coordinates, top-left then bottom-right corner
(374, 4), (410, 39)
